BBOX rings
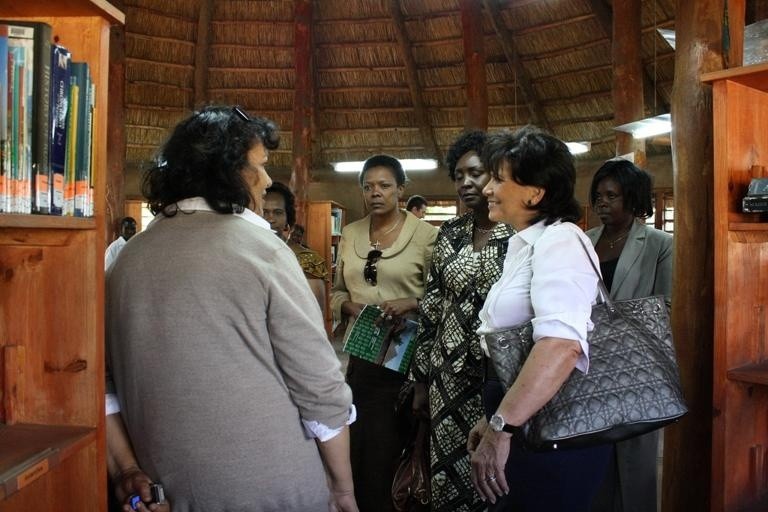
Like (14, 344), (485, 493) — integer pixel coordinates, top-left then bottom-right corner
(486, 477), (497, 481)
(376, 305), (393, 321)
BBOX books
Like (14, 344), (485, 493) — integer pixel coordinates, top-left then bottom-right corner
(340, 303), (420, 376)
(0, 19), (97, 218)
(332, 208), (344, 264)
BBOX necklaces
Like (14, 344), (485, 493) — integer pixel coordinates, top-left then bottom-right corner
(285, 233), (291, 244)
(474, 224), (493, 234)
(370, 218), (406, 249)
(602, 229), (630, 250)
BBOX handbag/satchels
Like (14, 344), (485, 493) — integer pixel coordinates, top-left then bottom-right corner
(391, 417), (431, 512)
(484, 231), (689, 453)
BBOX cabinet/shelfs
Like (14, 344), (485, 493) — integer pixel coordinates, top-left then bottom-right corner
(399, 194), (460, 229)
(125, 198), (159, 233)
(306, 199), (348, 339)
(0, 0), (126, 512)
(696, 59), (768, 512)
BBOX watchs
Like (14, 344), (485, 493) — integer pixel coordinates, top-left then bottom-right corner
(487, 414), (522, 435)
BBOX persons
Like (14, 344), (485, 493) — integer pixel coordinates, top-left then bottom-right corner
(104, 105), (357, 511)
(582, 159), (675, 511)
(467, 122), (606, 511)
(293, 224), (310, 249)
(331, 148), (440, 511)
(408, 195), (429, 219)
(263, 184), (329, 330)
(412, 128), (509, 512)
(105, 215), (139, 274)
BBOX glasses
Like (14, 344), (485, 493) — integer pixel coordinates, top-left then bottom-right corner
(363, 249), (382, 282)
(227, 106), (251, 138)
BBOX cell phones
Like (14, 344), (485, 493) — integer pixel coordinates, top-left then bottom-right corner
(126, 483), (166, 511)
